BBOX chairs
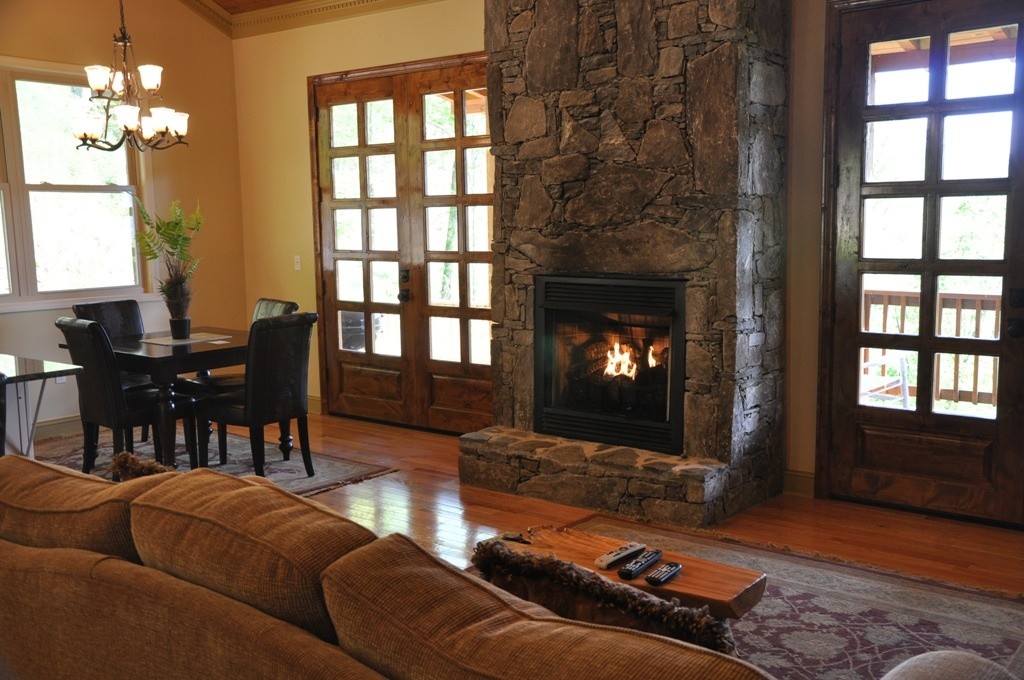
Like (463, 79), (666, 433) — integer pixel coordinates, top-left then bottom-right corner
(197, 312), (319, 478)
(55, 316), (195, 474)
(73, 299), (184, 447)
(183, 300), (300, 459)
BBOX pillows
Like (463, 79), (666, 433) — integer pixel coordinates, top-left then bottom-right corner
(472, 538), (739, 658)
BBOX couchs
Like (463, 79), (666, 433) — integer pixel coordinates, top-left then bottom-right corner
(1, 450), (1014, 680)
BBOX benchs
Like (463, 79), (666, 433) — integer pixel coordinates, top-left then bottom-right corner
(486, 524), (768, 618)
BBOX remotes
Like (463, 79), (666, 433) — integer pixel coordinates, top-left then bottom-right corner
(644, 560), (682, 587)
(594, 541), (653, 571)
(617, 547), (664, 581)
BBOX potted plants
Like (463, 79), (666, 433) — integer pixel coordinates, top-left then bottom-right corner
(132, 192), (204, 339)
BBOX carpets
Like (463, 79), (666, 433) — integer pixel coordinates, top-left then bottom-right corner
(34, 430), (399, 497)
(465, 505), (1024, 680)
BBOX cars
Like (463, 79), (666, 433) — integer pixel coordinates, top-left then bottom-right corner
(342, 309), (382, 351)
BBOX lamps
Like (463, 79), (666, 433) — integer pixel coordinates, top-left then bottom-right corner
(75, 0), (189, 151)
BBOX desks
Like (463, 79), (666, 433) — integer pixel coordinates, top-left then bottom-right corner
(58, 325), (293, 470)
(0, 353), (85, 456)
